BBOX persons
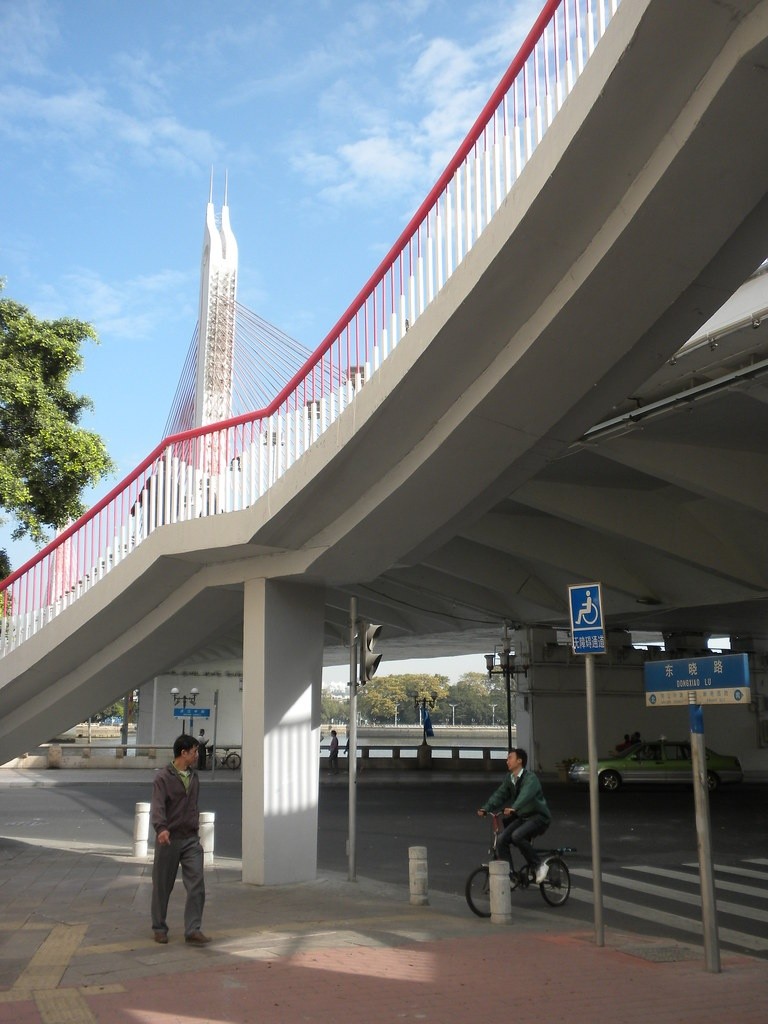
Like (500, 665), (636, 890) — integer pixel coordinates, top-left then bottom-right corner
(612, 731), (655, 761)
(327, 730), (339, 774)
(477, 748), (550, 884)
(197, 729), (210, 771)
(150, 733), (211, 943)
(343, 738), (349, 769)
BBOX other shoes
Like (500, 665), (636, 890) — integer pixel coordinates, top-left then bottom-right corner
(328, 771), (339, 776)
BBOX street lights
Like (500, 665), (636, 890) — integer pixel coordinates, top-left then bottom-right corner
(170, 687), (200, 735)
(484, 624), (531, 758)
(488, 699), (498, 726)
(392, 702), (401, 725)
(449, 703), (460, 726)
(410, 690), (438, 744)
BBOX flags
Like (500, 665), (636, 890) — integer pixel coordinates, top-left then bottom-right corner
(422, 707), (434, 738)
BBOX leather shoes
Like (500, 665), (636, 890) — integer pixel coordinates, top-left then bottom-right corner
(155, 926), (168, 943)
(186, 932), (210, 943)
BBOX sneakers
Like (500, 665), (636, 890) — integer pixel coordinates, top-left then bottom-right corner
(536, 863), (549, 884)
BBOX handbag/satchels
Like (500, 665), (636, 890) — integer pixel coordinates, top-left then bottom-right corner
(502, 814), (518, 828)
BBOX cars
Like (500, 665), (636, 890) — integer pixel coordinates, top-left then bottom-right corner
(566, 735), (743, 792)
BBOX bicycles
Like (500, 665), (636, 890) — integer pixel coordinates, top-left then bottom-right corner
(464, 808), (578, 917)
(206, 745), (242, 770)
